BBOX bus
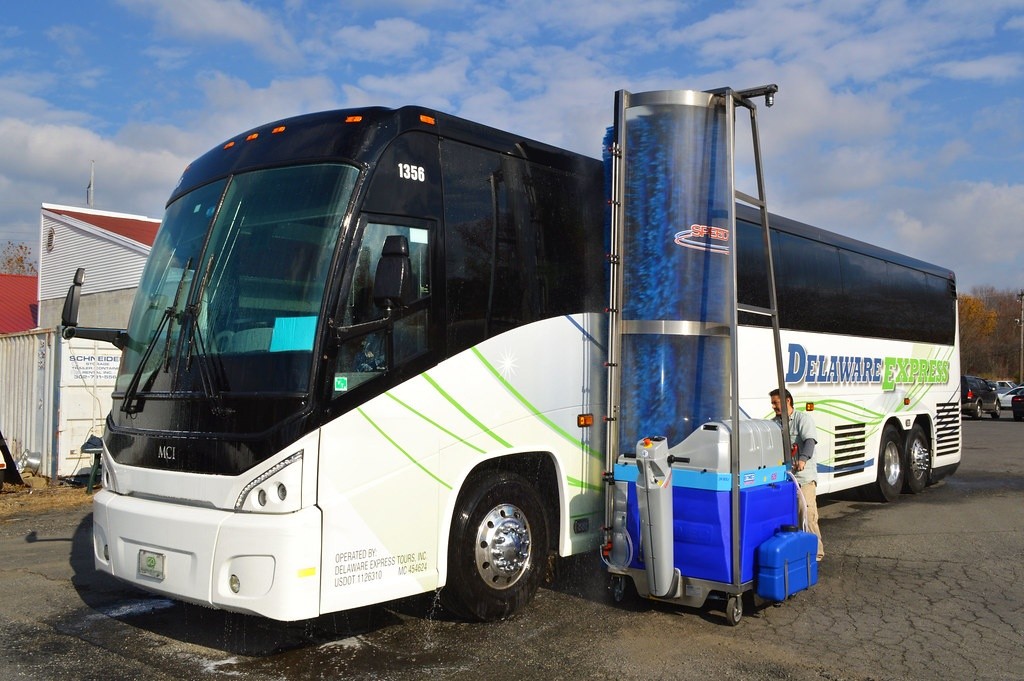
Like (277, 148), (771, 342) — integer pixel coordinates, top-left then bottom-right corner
(59, 105), (963, 622)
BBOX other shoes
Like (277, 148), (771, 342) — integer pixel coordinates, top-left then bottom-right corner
(816, 555), (823, 561)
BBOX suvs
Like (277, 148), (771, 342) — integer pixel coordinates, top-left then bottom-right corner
(960, 376), (1002, 419)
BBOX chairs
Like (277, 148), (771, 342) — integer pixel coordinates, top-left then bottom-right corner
(371, 234), (414, 314)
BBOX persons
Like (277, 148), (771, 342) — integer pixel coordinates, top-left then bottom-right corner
(769, 387), (825, 562)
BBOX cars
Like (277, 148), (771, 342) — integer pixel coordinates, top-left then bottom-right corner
(1011, 389), (1024, 421)
(983, 380), (1024, 408)
